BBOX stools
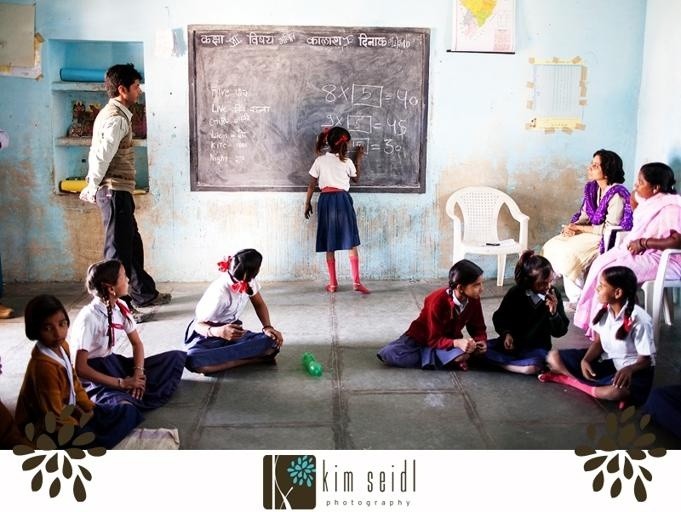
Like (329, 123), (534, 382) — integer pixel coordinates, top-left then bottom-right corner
(636, 244), (680, 337)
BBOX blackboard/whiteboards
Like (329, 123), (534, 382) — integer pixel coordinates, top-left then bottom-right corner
(187, 24), (430, 193)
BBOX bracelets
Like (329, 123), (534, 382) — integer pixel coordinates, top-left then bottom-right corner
(583, 225), (585, 234)
(644, 237), (653, 249)
(262, 326), (276, 332)
(132, 366), (146, 371)
(118, 378), (122, 390)
(639, 238), (646, 249)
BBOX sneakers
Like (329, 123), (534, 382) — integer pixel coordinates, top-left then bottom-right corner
(354, 282), (368, 293)
(131, 309), (153, 324)
(326, 284), (337, 292)
(136, 293), (170, 308)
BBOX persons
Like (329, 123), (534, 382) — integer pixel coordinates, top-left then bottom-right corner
(185, 248), (284, 374)
(15, 294), (145, 450)
(544, 149), (633, 313)
(303, 127), (371, 293)
(573, 162), (681, 341)
(69, 259), (187, 414)
(467, 251), (570, 376)
(536, 266), (656, 412)
(377, 258), (488, 371)
(78, 64), (172, 323)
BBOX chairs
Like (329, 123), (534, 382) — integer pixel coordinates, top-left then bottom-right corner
(604, 224), (671, 326)
(447, 183), (534, 288)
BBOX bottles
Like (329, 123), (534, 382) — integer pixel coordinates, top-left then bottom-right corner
(301, 352), (323, 379)
(81, 159), (88, 180)
